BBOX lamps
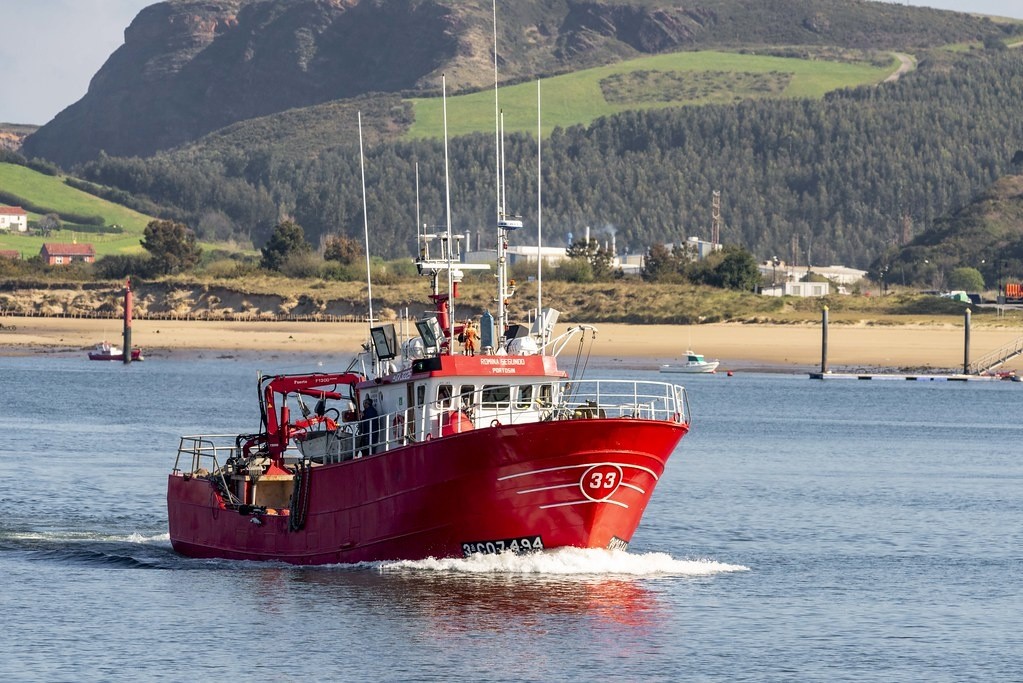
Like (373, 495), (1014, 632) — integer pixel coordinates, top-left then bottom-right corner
(371, 323), (402, 361)
(416, 316), (445, 358)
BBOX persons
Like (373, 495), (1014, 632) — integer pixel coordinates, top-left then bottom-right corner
(360, 399), (379, 457)
(464, 321), (480, 357)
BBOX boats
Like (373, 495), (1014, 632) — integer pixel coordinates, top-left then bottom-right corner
(166, 0), (692, 567)
(86, 341), (141, 361)
(660, 351), (721, 373)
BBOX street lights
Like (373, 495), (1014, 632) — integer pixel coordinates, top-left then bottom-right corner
(981, 254), (1008, 304)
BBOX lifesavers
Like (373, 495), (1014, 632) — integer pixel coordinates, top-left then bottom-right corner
(392, 415), (405, 444)
